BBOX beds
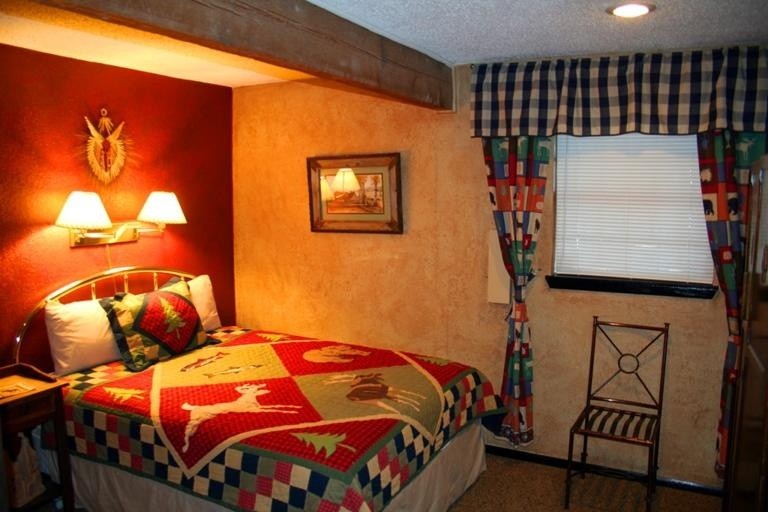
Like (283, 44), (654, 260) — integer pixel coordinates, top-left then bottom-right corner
(11, 265), (507, 511)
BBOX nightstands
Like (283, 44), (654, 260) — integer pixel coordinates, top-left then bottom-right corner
(0, 363), (74, 512)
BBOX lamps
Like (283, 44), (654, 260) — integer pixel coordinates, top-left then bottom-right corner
(55, 190), (187, 249)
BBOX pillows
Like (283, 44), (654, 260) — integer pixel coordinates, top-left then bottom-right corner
(101, 277), (219, 373)
(186, 272), (224, 333)
(43, 297), (120, 376)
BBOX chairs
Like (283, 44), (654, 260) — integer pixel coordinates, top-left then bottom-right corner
(566, 315), (670, 512)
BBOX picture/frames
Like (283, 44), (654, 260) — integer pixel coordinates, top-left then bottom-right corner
(308, 152), (403, 234)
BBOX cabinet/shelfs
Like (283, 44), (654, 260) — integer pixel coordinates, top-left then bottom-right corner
(723, 337), (767, 511)
